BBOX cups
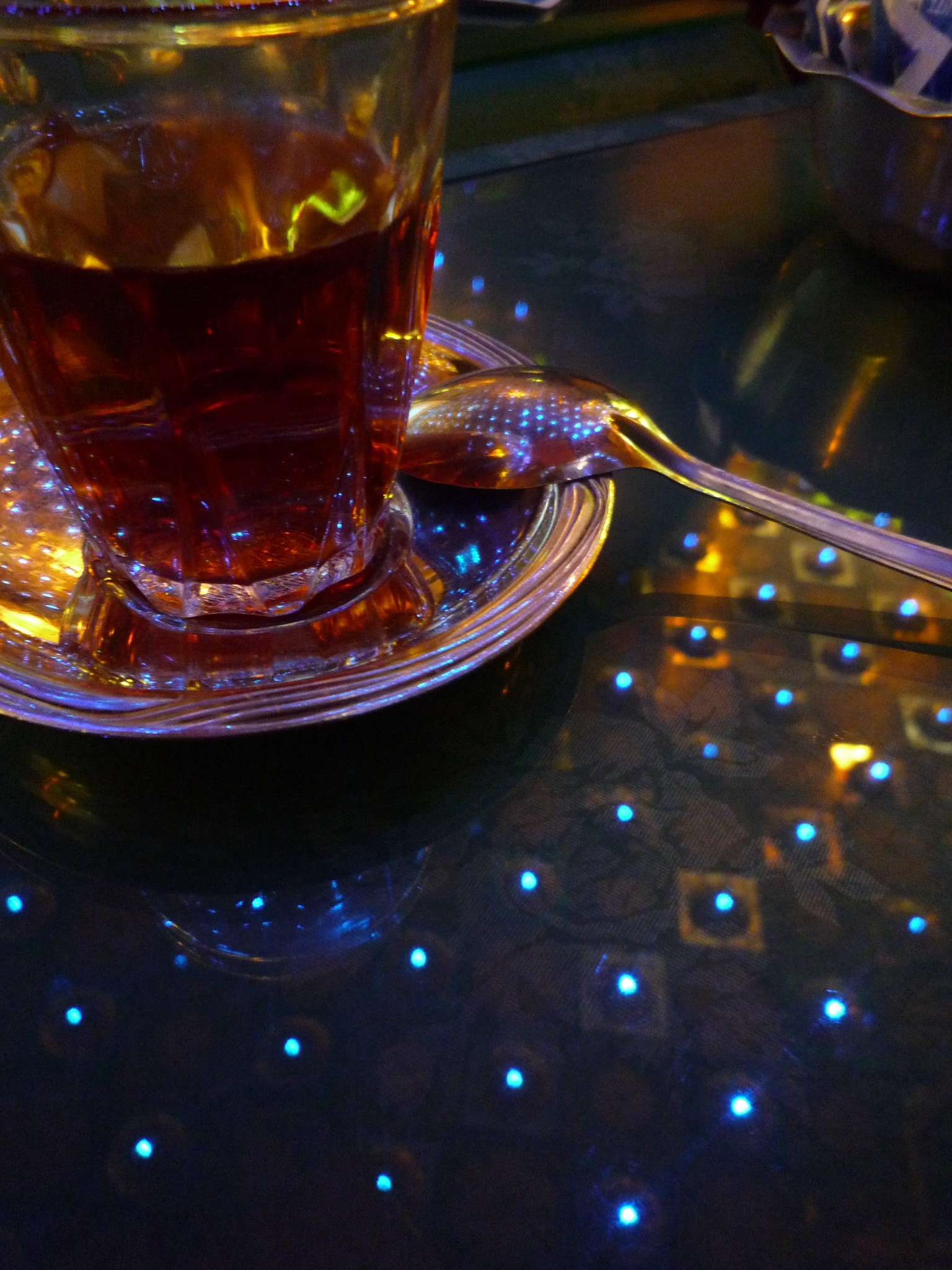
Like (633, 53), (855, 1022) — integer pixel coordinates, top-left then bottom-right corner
(0, 2), (455, 684)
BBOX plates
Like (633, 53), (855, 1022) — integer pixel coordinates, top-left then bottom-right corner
(1, 370), (614, 739)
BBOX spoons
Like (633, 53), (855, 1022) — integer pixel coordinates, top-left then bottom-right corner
(402, 365), (952, 592)
